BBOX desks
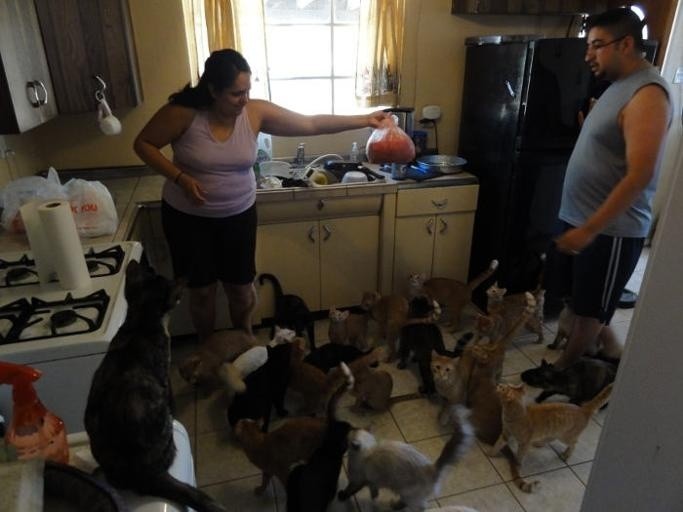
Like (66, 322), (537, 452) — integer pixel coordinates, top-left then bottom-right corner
(1, 420), (196, 512)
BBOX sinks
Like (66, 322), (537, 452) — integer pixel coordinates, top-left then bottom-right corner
(305, 164), (384, 188)
(254, 167), (310, 191)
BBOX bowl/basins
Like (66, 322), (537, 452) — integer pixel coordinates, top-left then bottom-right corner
(260, 160), (292, 178)
(416, 155), (467, 173)
(339, 171), (369, 184)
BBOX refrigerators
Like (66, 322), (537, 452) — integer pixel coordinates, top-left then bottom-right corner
(460, 34), (659, 319)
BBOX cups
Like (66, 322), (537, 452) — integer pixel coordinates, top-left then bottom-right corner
(392, 162), (408, 180)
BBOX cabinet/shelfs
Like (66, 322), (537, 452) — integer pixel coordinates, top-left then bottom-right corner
(451, 1), (611, 16)
(34, 2), (143, 114)
(387, 175), (480, 310)
(130, 190), (381, 331)
(0, 0), (58, 136)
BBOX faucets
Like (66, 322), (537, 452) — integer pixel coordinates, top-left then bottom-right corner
(297, 144), (304, 165)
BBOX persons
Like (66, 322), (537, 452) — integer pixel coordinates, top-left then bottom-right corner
(520, 8), (675, 388)
(134, 49), (386, 345)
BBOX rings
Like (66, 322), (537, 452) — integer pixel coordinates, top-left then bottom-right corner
(571, 249), (579, 254)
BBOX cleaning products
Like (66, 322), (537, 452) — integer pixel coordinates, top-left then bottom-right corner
(0, 361), (68, 465)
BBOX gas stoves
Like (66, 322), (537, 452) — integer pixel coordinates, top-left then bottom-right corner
(0, 241), (142, 364)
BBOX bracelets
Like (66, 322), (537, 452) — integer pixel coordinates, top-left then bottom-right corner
(174, 171), (183, 184)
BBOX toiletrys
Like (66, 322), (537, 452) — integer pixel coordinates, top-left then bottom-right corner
(350, 142), (360, 163)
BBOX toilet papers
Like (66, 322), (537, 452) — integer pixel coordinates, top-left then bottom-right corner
(20, 199), (90, 289)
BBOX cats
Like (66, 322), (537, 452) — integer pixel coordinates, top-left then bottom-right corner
(335, 409), (469, 511)
(348, 258), (627, 442)
(487, 383), (622, 462)
(84, 258), (228, 512)
(181, 260), (348, 512)
